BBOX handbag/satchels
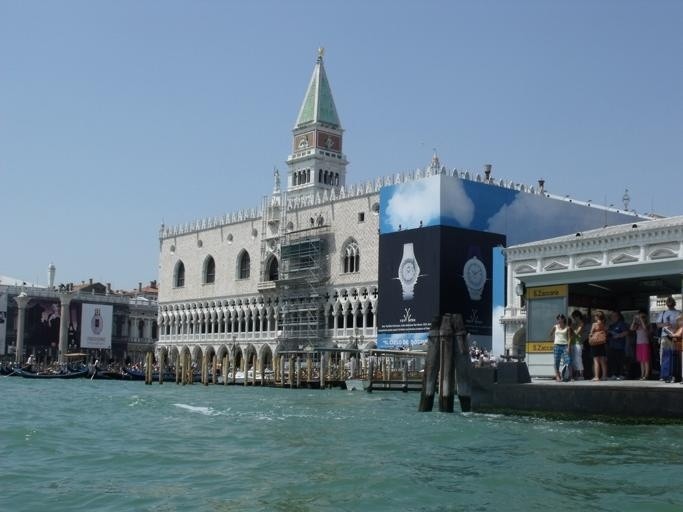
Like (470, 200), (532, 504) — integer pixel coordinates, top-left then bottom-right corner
(658, 311), (665, 337)
(589, 331), (607, 346)
(561, 364), (570, 382)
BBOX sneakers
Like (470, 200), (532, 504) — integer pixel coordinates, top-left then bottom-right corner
(639, 376), (648, 380)
(591, 375), (627, 381)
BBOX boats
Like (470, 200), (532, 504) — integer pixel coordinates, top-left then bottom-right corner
(218, 372), (273, 384)
(0, 360), (212, 383)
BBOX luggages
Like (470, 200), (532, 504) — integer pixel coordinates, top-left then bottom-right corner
(661, 342), (682, 382)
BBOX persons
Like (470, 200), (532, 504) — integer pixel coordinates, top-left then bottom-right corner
(468, 339), (479, 354)
(547, 295), (682, 383)
(94, 356), (101, 368)
(478, 345), (489, 363)
(273, 165), (279, 190)
(28, 355), (34, 365)
(315, 213), (324, 227)
(308, 216), (314, 228)
(47, 341), (57, 366)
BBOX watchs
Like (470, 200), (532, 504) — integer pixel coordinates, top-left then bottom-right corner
(397, 242), (420, 302)
(461, 254), (486, 300)
